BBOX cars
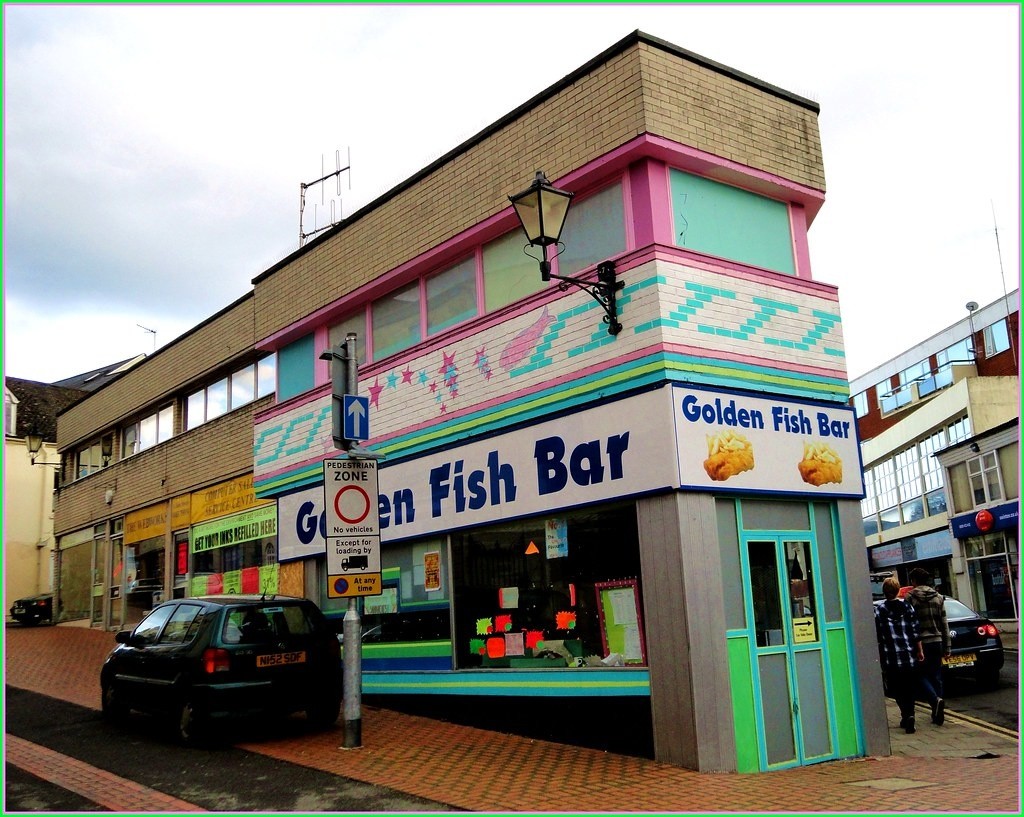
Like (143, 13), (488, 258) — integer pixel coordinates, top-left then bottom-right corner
(100, 593), (344, 748)
(873, 595), (1004, 697)
(10, 593), (52, 625)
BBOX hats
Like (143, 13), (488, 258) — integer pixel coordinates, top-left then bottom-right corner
(908, 568), (928, 581)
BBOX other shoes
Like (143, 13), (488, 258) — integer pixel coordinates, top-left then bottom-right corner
(937, 697), (944, 726)
(931, 710), (937, 722)
(906, 716), (916, 734)
(900, 718), (907, 728)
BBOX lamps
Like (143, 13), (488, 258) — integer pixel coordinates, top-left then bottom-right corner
(24, 423), (66, 468)
(505, 171), (625, 335)
(80, 438), (115, 469)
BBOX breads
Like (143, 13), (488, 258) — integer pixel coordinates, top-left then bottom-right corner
(798, 442), (843, 486)
(703, 430), (755, 481)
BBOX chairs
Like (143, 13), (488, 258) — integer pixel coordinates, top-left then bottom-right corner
(240, 612), (277, 642)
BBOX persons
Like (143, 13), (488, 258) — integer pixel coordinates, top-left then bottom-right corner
(904, 568), (952, 725)
(873, 577), (925, 734)
